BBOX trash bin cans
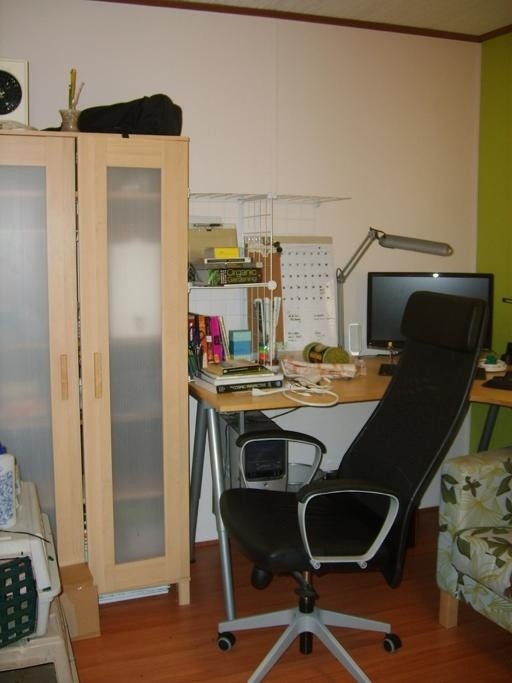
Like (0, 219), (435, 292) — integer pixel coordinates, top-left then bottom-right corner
(287, 462), (324, 492)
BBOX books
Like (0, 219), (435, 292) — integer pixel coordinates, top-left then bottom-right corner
(188, 311), (231, 377)
(192, 362), (283, 395)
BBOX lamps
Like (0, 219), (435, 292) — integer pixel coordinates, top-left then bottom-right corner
(336, 226), (453, 350)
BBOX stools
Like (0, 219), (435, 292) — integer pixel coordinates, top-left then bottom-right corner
(0, 598), (80, 683)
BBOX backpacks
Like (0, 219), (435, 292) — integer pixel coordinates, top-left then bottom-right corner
(78, 93), (183, 138)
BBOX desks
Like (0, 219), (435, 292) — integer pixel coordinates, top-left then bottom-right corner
(189, 355), (512, 620)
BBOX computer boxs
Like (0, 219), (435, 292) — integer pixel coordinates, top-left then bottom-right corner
(210, 411), (289, 517)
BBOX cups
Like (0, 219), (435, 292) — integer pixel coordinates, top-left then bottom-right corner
(349, 324), (363, 356)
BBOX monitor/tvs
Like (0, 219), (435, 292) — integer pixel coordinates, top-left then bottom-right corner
(366, 271), (494, 351)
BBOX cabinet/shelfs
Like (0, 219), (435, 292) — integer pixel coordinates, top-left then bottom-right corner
(0, 129), (192, 606)
(188, 190), (351, 373)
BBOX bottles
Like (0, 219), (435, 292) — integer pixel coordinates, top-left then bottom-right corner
(0, 447), (17, 531)
(302, 342), (347, 365)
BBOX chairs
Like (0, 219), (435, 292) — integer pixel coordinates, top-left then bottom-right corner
(216, 292), (489, 683)
(435, 445), (512, 633)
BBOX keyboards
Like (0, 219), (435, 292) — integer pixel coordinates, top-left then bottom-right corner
(378, 362), (486, 380)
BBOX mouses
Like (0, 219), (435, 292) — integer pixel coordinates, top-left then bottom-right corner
(503, 369), (512, 386)
